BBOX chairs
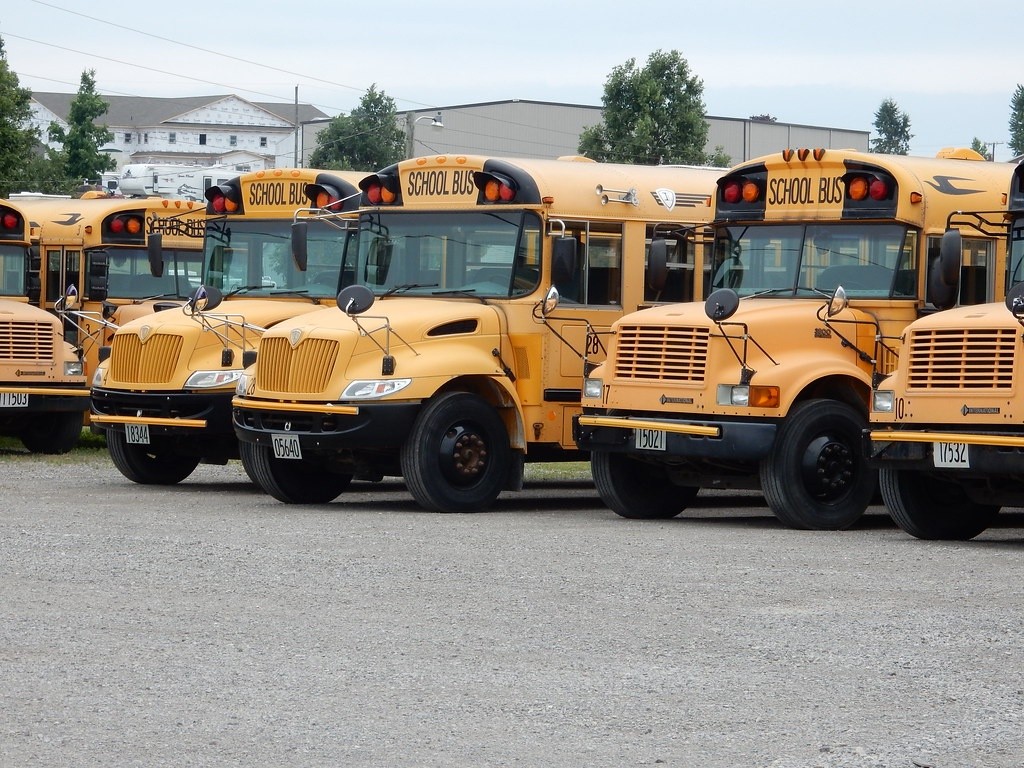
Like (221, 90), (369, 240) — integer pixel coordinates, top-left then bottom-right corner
(474, 267), (538, 293)
(817, 263), (892, 292)
(139, 273), (192, 297)
(317, 270), (354, 287)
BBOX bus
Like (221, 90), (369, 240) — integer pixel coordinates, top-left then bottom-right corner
(53, 164), (784, 487)
(532, 136), (1024, 532)
(817, 151), (1022, 541)
(1, 182), (268, 456)
(182, 141), (986, 515)
